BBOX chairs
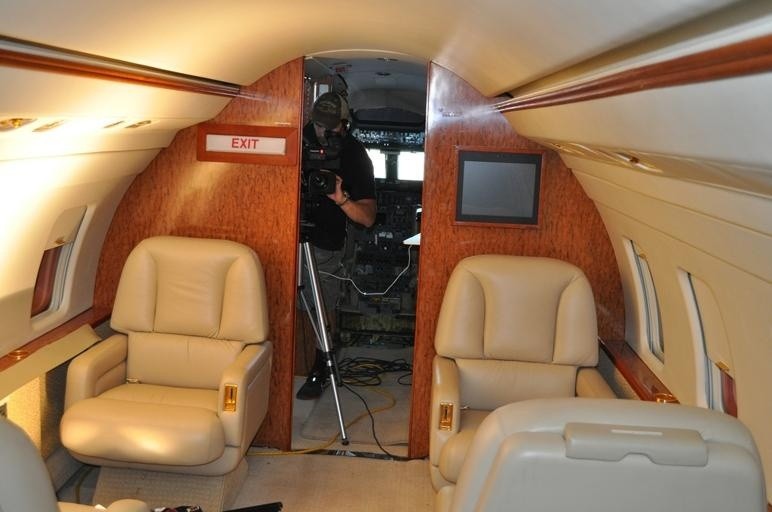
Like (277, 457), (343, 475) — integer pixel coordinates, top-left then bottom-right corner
(436, 398), (767, 512)
(426, 254), (618, 493)
(56, 235), (273, 512)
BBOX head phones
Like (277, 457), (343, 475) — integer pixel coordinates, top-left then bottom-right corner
(338, 93), (353, 131)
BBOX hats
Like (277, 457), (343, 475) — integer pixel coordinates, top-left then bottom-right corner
(310, 92), (352, 127)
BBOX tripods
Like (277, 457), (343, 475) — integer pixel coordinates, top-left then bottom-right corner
(298, 215), (349, 446)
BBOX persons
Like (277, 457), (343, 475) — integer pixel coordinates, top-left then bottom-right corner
(297, 92), (378, 399)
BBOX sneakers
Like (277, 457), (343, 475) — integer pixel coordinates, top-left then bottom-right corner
(297, 373), (326, 399)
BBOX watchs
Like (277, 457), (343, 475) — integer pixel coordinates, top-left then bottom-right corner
(337, 190), (350, 207)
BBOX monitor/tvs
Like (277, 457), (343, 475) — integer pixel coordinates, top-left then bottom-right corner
(456, 150), (542, 224)
